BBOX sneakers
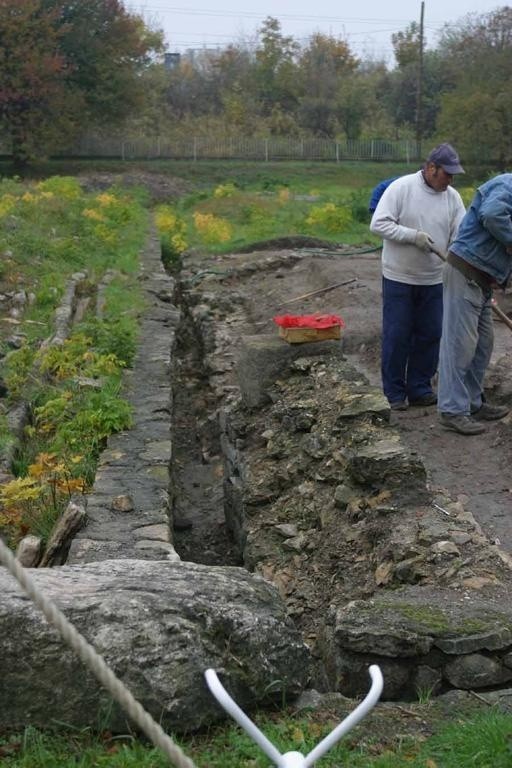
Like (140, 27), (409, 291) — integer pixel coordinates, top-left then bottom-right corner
(471, 401), (509, 422)
(437, 409), (486, 436)
(408, 391), (437, 407)
(388, 400), (408, 412)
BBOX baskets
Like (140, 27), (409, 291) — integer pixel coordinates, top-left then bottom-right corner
(277, 320), (341, 344)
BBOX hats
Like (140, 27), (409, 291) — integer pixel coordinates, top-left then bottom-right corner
(427, 141), (466, 176)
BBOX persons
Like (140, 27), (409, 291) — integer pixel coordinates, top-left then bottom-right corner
(369, 145), (466, 410)
(437, 173), (512, 435)
